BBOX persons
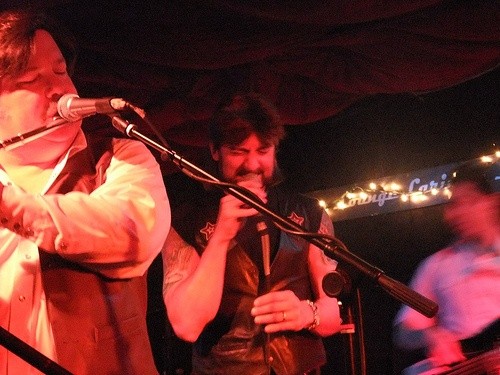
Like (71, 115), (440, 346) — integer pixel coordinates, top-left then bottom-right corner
(0, 10), (172, 375)
(161, 93), (341, 375)
(393, 168), (500, 375)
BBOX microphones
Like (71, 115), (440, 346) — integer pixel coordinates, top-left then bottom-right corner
(56, 92), (129, 124)
(242, 172), (272, 277)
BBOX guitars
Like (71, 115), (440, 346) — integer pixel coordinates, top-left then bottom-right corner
(399, 348), (500, 375)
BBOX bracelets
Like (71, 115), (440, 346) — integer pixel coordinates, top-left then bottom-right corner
(303, 298), (319, 331)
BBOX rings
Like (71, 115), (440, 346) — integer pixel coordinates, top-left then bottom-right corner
(282, 312), (285, 321)
